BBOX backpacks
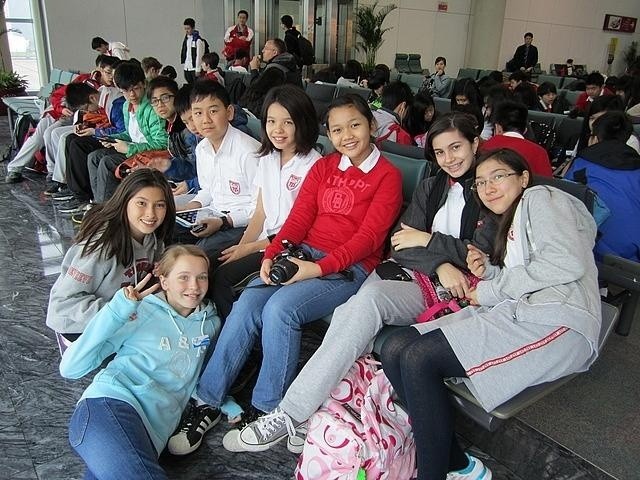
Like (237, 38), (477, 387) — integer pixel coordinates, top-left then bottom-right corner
(296, 35), (314, 66)
(295, 355), (417, 479)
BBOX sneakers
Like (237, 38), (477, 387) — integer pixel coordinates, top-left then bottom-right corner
(445, 457), (492, 480)
(168, 396), (309, 455)
(6, 170), (92, 223)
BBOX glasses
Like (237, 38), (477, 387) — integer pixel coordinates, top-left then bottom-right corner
(470, 171), (520, 193)
(150, 95), (174, 106)
(120, 85), (142, 94)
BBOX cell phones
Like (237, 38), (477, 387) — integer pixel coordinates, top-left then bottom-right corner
(376, 261), (412, 282)
(192, 223), (207, 233)
(167, 180), (180, 192)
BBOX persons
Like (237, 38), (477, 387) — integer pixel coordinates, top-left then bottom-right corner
(43, 165), (176, 358)
(167, 92), (402, 458)
(195, 83), (326, 396)
(5, 11), (303, 224)
(380, 147), (605, 479)
(312, 32), (639, 259)
(59, 242), (224, 479)
(237, 113), (483, 454)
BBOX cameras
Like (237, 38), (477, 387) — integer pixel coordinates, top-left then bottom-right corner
(270, 239), (315, 285)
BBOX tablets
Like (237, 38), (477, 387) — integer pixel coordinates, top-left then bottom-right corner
(176, 207), (225, 228)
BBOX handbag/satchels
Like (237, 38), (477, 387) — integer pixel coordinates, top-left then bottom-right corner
(23, 127), (46, 171)
(414, 269), (480, 322)
(115, 150), (171, 179)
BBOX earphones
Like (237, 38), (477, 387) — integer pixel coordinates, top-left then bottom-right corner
(401, 108), (404, 114)
